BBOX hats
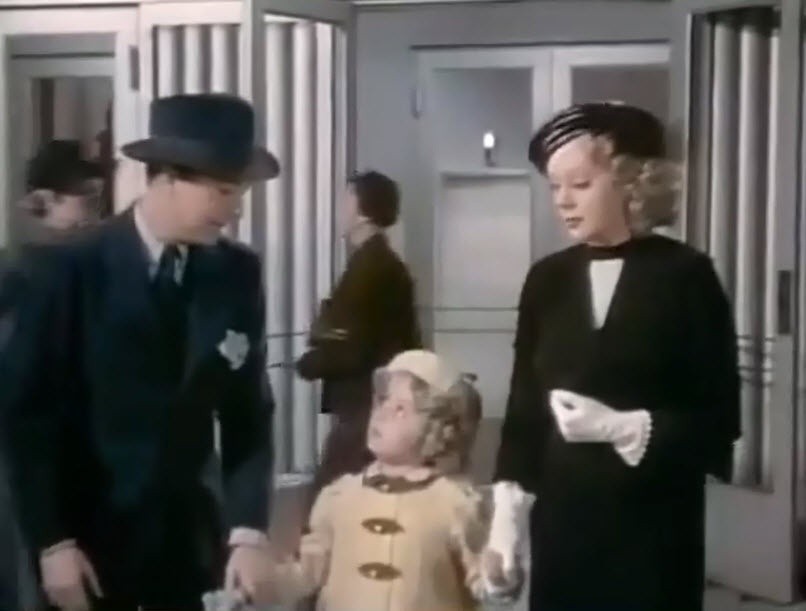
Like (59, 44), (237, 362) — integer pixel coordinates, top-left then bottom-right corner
(121, 93), (278, 181)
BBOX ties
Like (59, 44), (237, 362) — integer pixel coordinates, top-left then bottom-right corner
(149, 246), (182, 385)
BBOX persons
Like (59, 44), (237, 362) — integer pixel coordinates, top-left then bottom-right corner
(202, 348), (525, 610)
(0, 140), (108, 611)
(291, 171), (417, 526)
(478, 100), (740, 611)
(0, 93), (275, 611)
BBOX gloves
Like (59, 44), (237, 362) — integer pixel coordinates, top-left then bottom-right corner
(201, 590), (241, 611)
(40, 539), (102, 611)
(548, 389), (652, 467)
(479, 481), (536, 606)
(225, 545), (277, 611)
